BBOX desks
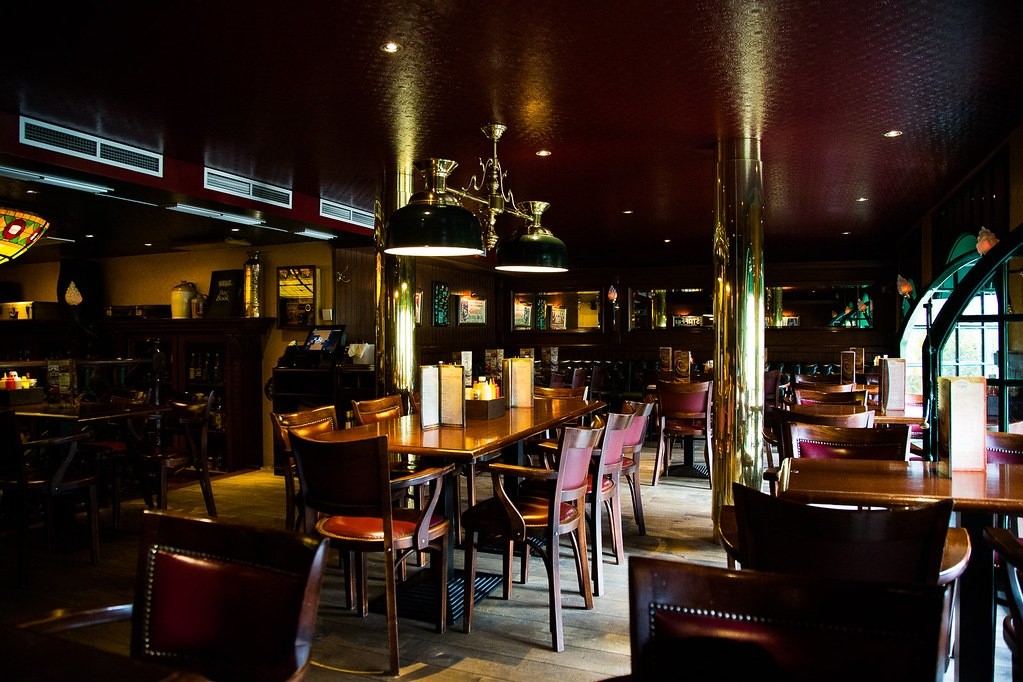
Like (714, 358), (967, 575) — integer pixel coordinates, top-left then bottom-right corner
(272, 365), (374, 478)
(776, 404), (925, 426)
(776, 456), (1023, 682)
(307, 396), (607, 611)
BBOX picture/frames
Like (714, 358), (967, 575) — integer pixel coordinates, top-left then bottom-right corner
(415, 287), (424, 327)
(550, 306), (567, 330)
(430, 279), (451, 327)
(455, 295), (487, 326)
(514, 300), (532, 330)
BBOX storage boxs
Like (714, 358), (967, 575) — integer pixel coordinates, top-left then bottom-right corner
(353, 344), (375, 365)
(104, 305), (172, 321)
(0, 301), (58, 320)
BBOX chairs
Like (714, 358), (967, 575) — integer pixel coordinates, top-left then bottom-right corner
(0, 388), (456, 682)
(462, 358), (1022, 682)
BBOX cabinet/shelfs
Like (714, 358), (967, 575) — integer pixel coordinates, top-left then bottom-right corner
(127, 334), (263, 473)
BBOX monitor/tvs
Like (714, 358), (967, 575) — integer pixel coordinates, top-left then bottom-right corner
(300, 325), (346, 354)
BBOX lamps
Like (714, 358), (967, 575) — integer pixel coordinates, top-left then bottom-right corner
(0, 206), (50, 265)
(896, 274), (915, 307)
(976, 226), (1000, 255)
(383, 123), (569, 272)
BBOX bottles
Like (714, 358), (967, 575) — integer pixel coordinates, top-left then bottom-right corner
(660, 315), (667, 327)
(171, 281), (197, 319)
(191, 293), (206, 318)
(470, 376), (500, 400)
(0, 373), (31, 390)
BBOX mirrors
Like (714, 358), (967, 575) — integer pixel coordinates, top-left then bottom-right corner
(511, 279), (881, 332)
